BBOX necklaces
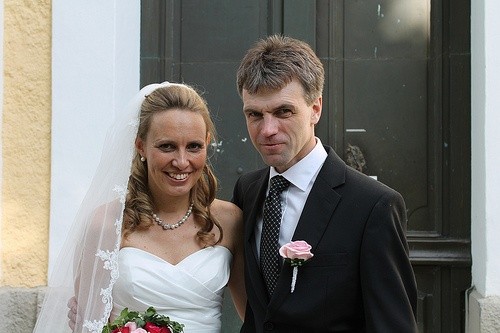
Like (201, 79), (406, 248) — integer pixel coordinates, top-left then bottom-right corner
(148, 198), (194, 231)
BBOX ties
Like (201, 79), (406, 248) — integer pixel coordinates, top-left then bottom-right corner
(260, 176), (290, 299)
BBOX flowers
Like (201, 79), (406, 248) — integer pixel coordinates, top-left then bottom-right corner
(279, 240), (314, 266)
(101, 306), (184, 333)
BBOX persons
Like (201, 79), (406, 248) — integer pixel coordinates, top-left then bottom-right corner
(72, 82), (248, 333)
(66, 35), (420, 333)
